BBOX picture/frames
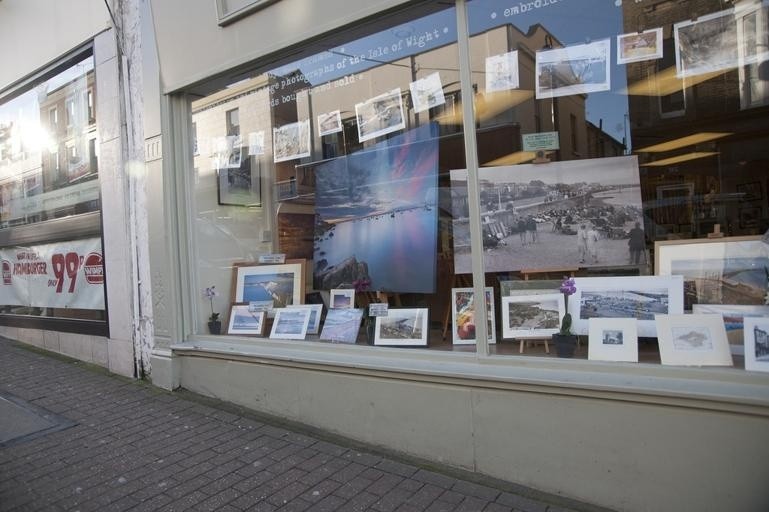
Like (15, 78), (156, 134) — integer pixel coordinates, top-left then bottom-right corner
(692, 303), (768, 355)
(225, 259), (323, 341)
(567, 277), (684, 337)
(653, 311), (735, 367)
(499, 280), (569, 341)
(741, 315), (768, 372)
(588, 317), (638, 365)
(451, 287), (498, 344)
(217, 154), (262, 207)
(369, 305), (431, 348)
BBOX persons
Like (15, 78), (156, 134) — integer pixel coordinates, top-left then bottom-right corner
(574, 224), (601, 265)
(624, 221), (646, 265)
(514, 215), (539, 246)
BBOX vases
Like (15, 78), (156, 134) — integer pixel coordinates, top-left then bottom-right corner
(207, 320), (221, 335)
(551, 333), (578, 358)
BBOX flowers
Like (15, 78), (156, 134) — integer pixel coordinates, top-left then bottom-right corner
(560, 279), (576, 334)
(202, 285), (220, 324)
